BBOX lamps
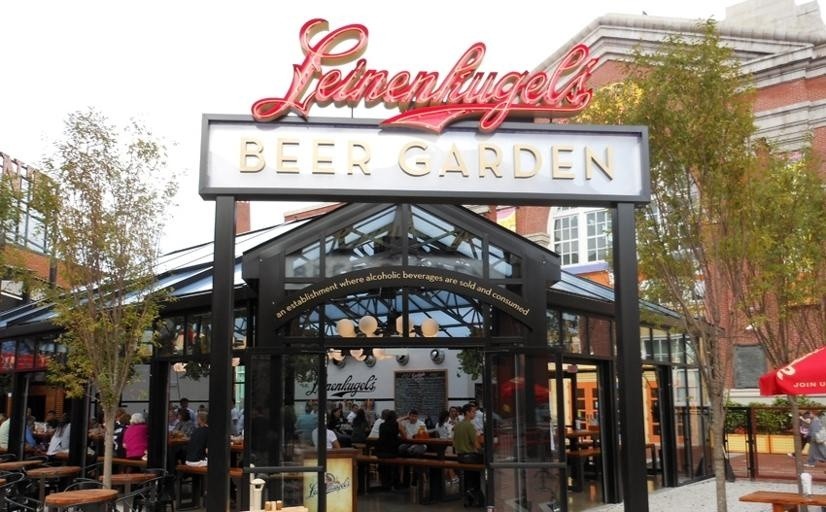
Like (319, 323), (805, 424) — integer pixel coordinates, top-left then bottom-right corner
(173, 315), (440, 372)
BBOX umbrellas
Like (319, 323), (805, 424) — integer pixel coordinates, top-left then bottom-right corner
(759, 345), (826, 398)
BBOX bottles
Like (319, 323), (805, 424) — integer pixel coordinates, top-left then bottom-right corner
(265, 499), (282, 511)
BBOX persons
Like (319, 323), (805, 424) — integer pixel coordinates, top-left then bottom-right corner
(167, 398), (209, 497)
(286, 398), (487, 501)
(787, 404), (812, 460)
(1, 406), (148, 474)
(803, 409), (825, 468)
(230, 398), (244, 468)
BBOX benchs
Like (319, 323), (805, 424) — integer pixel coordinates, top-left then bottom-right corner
(740, 491), (826, 512)
(567, 449), (599, 460)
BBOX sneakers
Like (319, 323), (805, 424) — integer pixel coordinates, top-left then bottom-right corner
(464, 488), (480, 507)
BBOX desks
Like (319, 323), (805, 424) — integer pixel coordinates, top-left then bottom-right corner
(565, 430), (601, 492)
(0, 426), (485, 512)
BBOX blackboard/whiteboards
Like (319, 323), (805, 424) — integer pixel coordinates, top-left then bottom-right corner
(394, 369), (448, 429)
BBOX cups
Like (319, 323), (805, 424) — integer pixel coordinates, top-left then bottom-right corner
(800, 472), (812, 496)
